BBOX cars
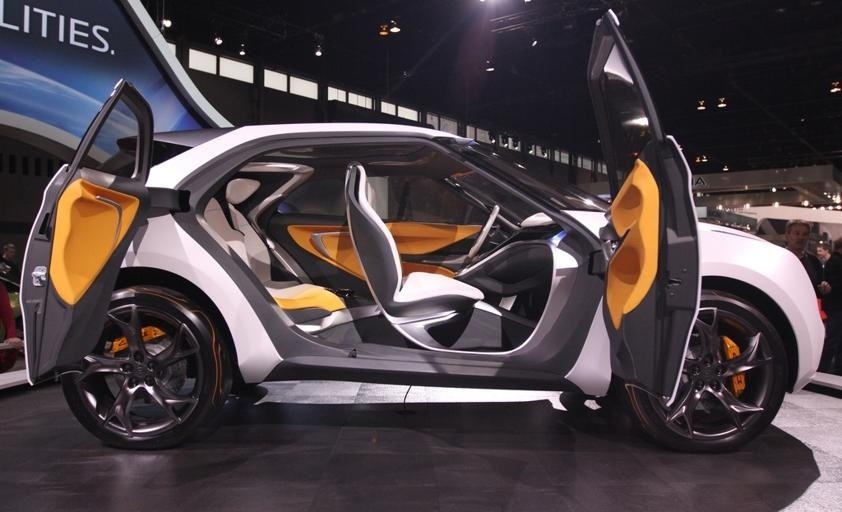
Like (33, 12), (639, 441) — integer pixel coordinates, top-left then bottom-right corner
(18, 4), (826, 459)
(0, 271), (23, 336)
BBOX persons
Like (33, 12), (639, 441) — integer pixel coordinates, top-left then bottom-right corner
(814, 241), (832, 270)
(0, 279), (25, 351)
(783, 218), (834, 375)
(821, 237), (842, 375)
(1, 242), (22, 294)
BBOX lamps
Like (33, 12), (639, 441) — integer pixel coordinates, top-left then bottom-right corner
(378, 16), (401, 37)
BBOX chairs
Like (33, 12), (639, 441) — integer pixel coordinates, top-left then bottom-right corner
(208, 173), (340, 318)
(348, 161), (486, 319)
